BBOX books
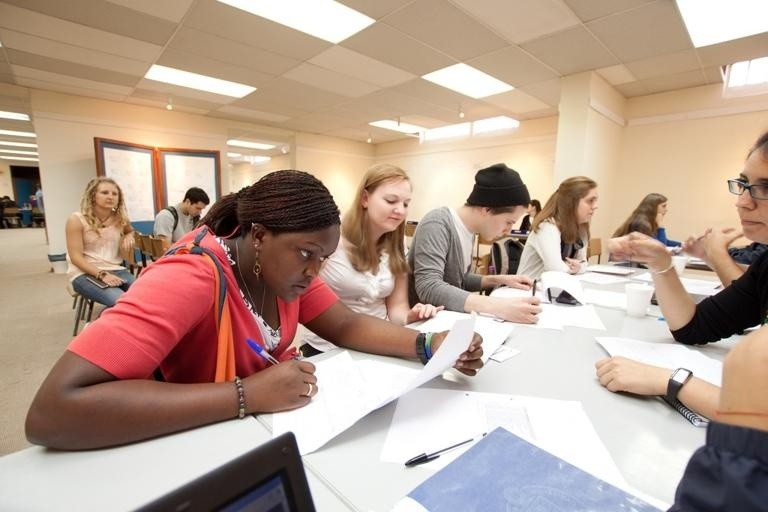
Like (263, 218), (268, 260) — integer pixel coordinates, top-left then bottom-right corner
(489, 269), (586, 307)
(650, 285), (713, 306)
(596, 334), (726, 430)
(685, 254), (713, 271)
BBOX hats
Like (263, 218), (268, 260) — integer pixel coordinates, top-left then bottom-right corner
(467, 164), (530, 207)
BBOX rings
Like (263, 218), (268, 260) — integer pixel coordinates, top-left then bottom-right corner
(306, 383), (312, 397)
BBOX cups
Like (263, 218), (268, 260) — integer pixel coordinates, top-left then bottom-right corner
(626, 282), (655, 316)
(671, 256), (687, 273)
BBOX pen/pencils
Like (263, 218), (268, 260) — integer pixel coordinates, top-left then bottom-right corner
(532, 279), (537, 305)
(405, 433), (487, 466)
(675, 235), (705, 254)
(247, 339), (279, 365)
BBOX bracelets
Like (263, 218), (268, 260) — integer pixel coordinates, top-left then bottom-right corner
(95, 269), (109, 281)
(425, 333), (433, 359)
(714, 410), (767, 417)
(416, 332), (428, 364)
(232, 375), (245, 419)
(647, 262), (675, 275)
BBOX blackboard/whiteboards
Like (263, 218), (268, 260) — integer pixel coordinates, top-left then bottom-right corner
(93, 136), (222, 223)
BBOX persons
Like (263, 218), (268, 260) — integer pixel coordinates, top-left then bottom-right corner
(408, 162), (542, 327)
(515, 176), (600, 277)
(667, 323), (767, 511)
(65, 176), (139, 307)
(520, 199), (541, 231)
(0, 181), (44, 229)
(594, 133), (768, 406)
(300, 163), (444, 358)
(609, 191), (686, 250)
(22, 169), (483, 450)
(682, 223), (768, 289)
(152, 186), (211, 246)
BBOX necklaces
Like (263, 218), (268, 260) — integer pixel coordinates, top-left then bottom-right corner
(235, 238), (266, 317)
(95, 215), (112, 229)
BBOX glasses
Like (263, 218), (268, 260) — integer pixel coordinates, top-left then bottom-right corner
(728, 178), (767, 199)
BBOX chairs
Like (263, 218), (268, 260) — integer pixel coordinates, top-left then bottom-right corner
(588, 238), (601, 264)
(2, 203), (45, 228)
(473, 231), (524, 297)
(66, 231), (164, 337)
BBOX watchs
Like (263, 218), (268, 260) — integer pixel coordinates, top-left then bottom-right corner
(665, 368), (693, 402)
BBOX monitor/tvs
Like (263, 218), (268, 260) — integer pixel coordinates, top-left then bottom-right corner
(130, 431), (316, 512)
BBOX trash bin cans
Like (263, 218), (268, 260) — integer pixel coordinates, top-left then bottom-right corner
(47, 252), (68, 274)
